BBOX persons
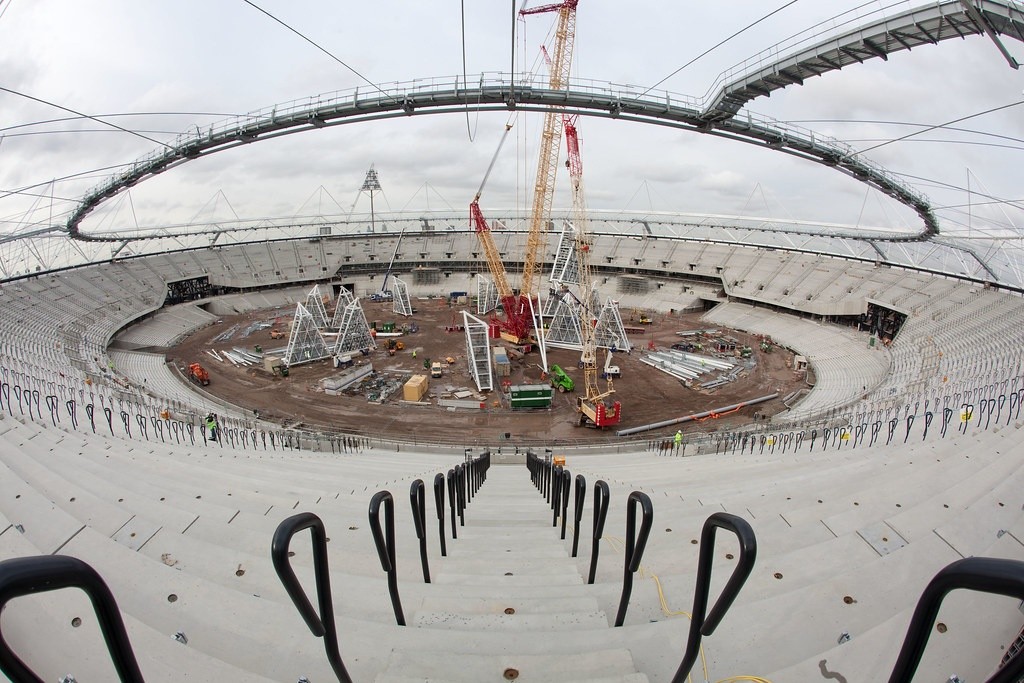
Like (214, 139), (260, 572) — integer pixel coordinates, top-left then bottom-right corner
(109, 361), (113, 369)
(372, 329), (377, 341)
(675, 430), (684, 449)
(753, 412), (759, 421)
(412, 350), (417, 359)
(702, 360), (704, 366)
(204, 412), (216, 441)
(698, 343), (702, 350)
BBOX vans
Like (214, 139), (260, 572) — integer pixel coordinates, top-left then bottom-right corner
(430, 362), (442, 379)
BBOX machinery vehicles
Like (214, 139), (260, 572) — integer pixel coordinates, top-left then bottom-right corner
(466, 0), (582, 356)
(536, 42), (623, 433)
(371, 226), (407, 303)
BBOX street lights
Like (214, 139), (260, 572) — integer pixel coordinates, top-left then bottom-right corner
(360, 165), (382, 233)
